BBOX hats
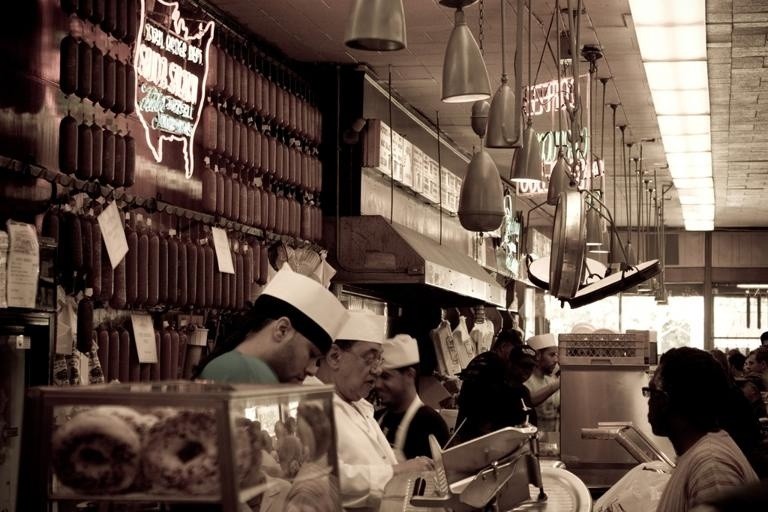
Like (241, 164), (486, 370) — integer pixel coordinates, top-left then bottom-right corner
(498, 328), (556, 366)
(259, 261), (420, 370)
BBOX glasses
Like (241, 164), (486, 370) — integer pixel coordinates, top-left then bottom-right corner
(642, 387), (666, 397)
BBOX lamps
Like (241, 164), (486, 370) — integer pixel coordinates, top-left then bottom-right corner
(548, 0), (674, 304)
(435, 1), (492, 104)
(341, 0), (410, 54)
(484, 1), (526, 153)
(458, 0), (506, 235)
(508, 1), (546, 186)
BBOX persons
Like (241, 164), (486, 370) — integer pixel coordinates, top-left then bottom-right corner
(300, 310), (435, 511)
(642, 346), (761, 511)
(235, 402), (339, 512)
(200, 263), (350, 390)
(369, 332), (450, 462)
(709, 331), (768, 439)
(452, 328), (562, 443)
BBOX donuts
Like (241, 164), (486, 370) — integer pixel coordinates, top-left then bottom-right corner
(51, 407), (250, 495)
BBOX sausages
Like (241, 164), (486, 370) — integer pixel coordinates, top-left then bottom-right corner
(60, 0), (324, 382)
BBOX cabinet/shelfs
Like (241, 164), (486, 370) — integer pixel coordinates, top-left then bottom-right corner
(16, 379), (344, 512)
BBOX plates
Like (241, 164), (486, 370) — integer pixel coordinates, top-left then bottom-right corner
(569, 322), (618, 334)
